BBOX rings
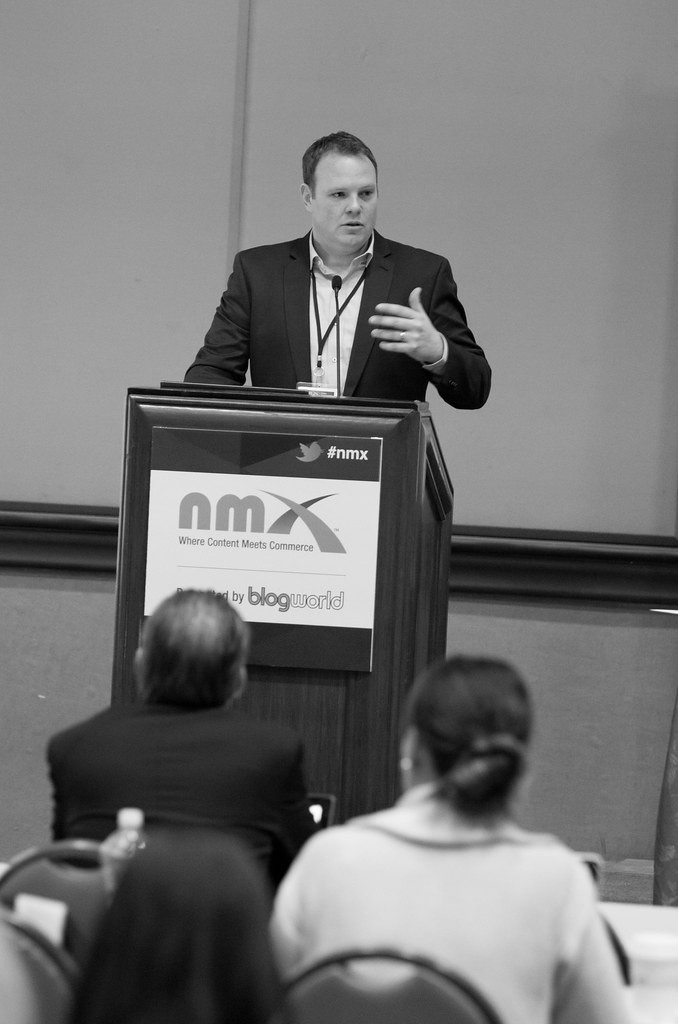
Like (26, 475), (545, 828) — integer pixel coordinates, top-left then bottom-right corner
(401, 332), (406, 341)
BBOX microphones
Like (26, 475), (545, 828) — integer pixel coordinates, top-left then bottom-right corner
(332, 274), (342, 399)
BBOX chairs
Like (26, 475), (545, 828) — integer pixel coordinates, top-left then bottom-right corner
(0, 793), (503, 1024)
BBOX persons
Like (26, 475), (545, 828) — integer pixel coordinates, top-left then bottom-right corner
(46, 588), (318, 898)
(184, 132), (493, 410)
(263, 656), (637, 1024)
(63, 824), (300, 1024)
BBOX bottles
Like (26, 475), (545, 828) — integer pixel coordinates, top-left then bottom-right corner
(99, 806), (147, 895)
